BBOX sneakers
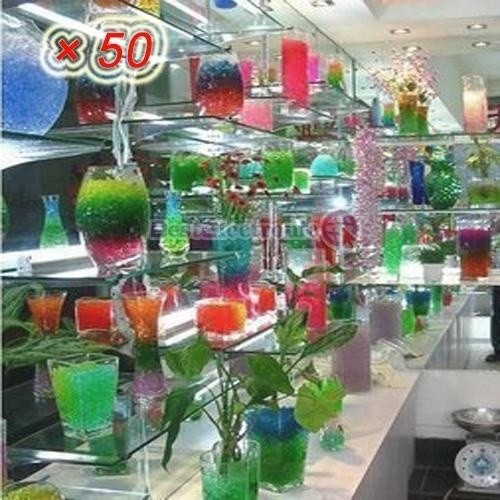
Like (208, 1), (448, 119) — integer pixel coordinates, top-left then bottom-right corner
(486, 355), (499, 361)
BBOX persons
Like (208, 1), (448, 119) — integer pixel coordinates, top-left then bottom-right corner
(484, 286), (500, 362)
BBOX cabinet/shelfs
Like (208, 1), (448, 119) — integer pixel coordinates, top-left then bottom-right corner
(1, 0), (499, 500)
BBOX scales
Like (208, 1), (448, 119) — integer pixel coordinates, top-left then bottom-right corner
(451, 406), (500, 494)
